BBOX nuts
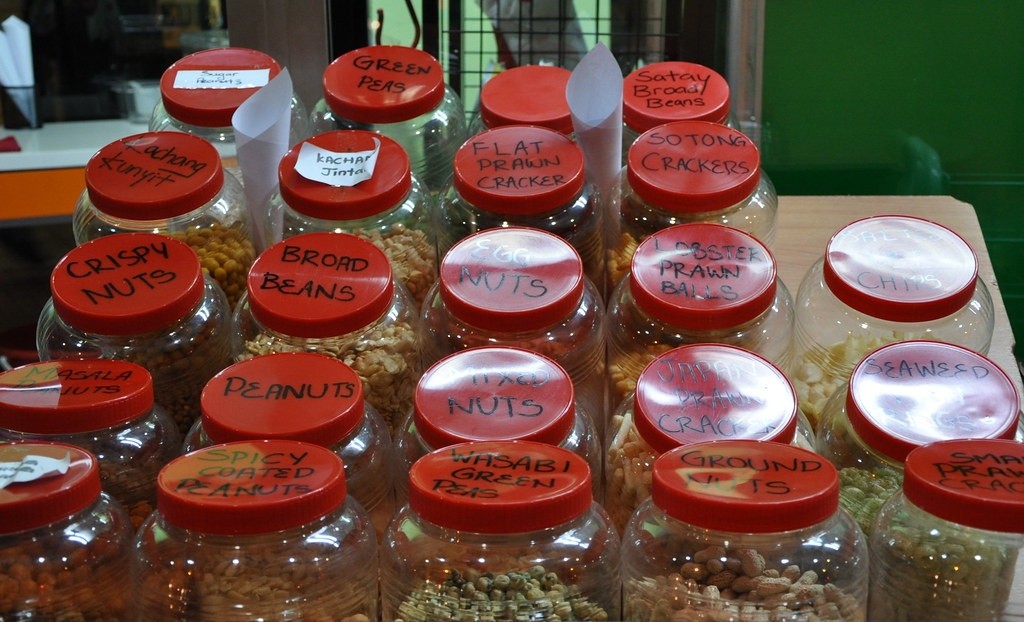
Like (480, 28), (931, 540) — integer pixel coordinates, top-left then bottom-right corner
(0, 190), (1024, 622)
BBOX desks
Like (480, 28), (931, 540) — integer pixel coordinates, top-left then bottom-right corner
(0, 118), (239, 230)
(723, 195), (1024, 414)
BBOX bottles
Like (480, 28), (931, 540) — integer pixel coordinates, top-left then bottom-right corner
(0, 47), (1024, 622)
